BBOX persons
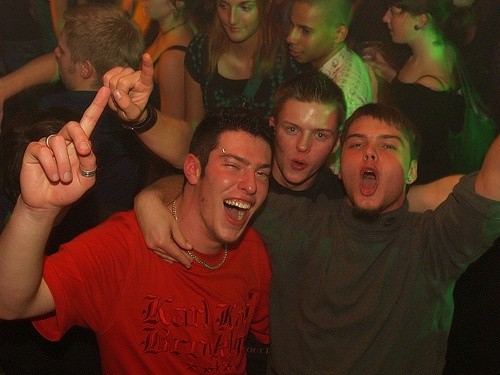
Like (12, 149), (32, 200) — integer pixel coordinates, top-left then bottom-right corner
(0, 85), (273, 375)
(103, 52), (469, 374)
(134, 103), (500, 375)
(0, 1), (500, 375)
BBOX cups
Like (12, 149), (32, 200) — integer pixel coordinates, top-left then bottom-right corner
(358, 41), (381, 66)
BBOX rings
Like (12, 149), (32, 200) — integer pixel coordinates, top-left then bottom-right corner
(80, 167), (97, 177)
(46, 133), (56, 147)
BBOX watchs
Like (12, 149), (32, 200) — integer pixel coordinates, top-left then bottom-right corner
(120, 103), (157, 136)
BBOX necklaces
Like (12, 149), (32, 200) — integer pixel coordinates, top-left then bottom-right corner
(172, 200), (227, 270)
(157, 20), (186, 41)
